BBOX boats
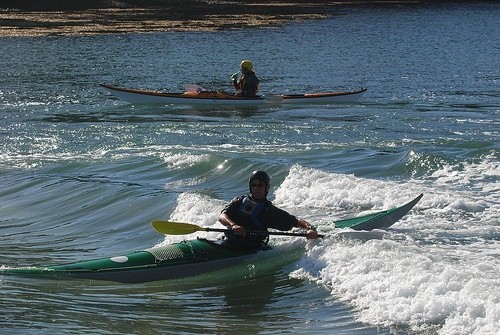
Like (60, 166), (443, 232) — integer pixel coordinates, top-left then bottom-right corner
(99, 82), (367, 105)
(0, 194), (424, 285)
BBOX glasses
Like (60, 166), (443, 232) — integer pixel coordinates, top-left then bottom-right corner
(250, 182), (265, 187)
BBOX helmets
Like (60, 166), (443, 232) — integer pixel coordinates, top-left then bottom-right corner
(248, 170), (270, 184)
(240, 60), (253, 71)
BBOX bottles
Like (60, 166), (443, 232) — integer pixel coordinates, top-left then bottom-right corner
(230, 70), (242, 81)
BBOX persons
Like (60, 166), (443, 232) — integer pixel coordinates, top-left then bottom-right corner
(218, 170), (318, 248)
(230, 60), (260, 97)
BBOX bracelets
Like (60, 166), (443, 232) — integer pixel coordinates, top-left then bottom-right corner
(306, 224), (317, 230)
(230, 223), (240, 229)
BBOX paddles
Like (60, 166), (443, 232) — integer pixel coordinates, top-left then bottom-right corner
(185, 83), (284, 103)
(152, 220), (383, 240)
